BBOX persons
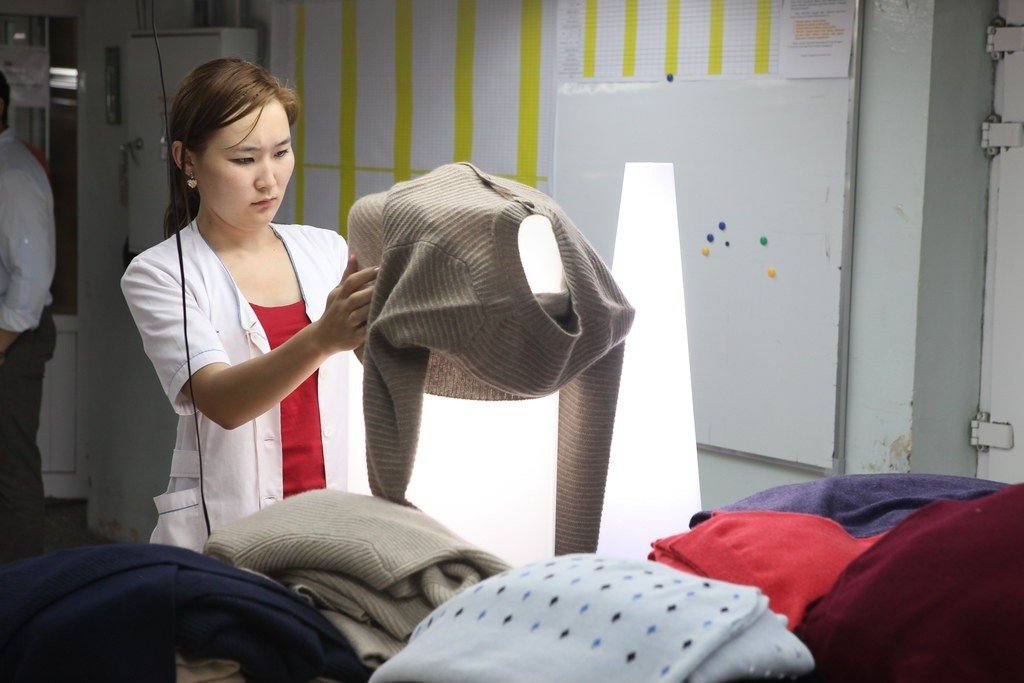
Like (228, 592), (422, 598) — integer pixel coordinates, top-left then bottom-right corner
(0, 69), (57, 566)
(121, 58), (380, 556)
